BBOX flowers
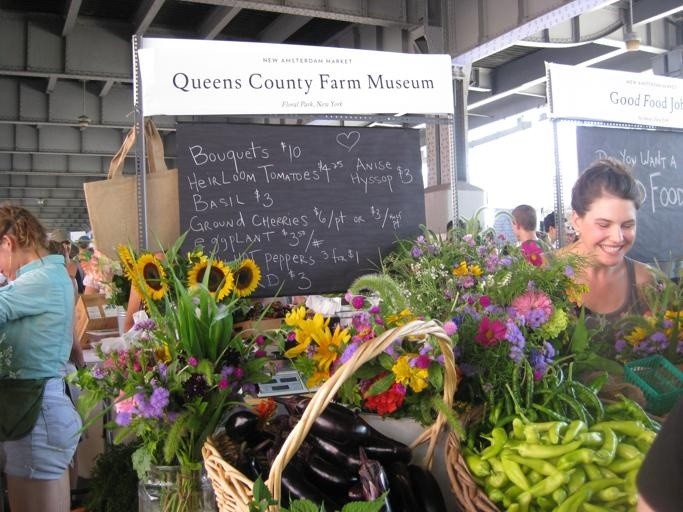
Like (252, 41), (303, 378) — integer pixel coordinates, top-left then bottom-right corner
(69, 227), (279, 476)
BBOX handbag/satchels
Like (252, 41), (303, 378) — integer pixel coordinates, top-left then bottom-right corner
(0, 377), (47, 439)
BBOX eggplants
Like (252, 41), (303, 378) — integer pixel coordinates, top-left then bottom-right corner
(226, 392), (445, 512)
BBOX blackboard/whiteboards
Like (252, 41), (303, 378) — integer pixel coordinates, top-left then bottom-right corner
(577, 125), (683, 263)
(177, 120), (427, 299)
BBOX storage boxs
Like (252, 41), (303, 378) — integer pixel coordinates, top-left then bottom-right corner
(74, 291), (119, 350)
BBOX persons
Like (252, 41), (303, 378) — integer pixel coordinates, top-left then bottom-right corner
(0, 202), (83, 511)
(529, 161), (683, 360)
(635, 391), (683, 512)
(447, 205), (568, 259)
(47, 227), (112, 512)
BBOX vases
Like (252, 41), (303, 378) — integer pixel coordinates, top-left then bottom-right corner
(137, 468), (213, 512)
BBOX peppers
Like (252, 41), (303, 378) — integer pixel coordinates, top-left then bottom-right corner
(461, 355), (665, 512)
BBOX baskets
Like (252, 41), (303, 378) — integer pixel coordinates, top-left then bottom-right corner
(449, 389), (661, 511)
(624, 356), (683, 413)
(202, 320), (500, 511)
